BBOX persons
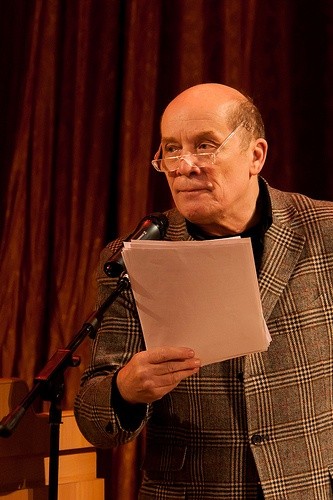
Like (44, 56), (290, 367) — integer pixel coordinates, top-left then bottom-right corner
(72, 83), (333, 499)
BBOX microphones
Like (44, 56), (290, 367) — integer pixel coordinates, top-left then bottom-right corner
(104, 212), (169, 277)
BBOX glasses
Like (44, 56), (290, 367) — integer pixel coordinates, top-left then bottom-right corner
(150, 122), (245, 172)
